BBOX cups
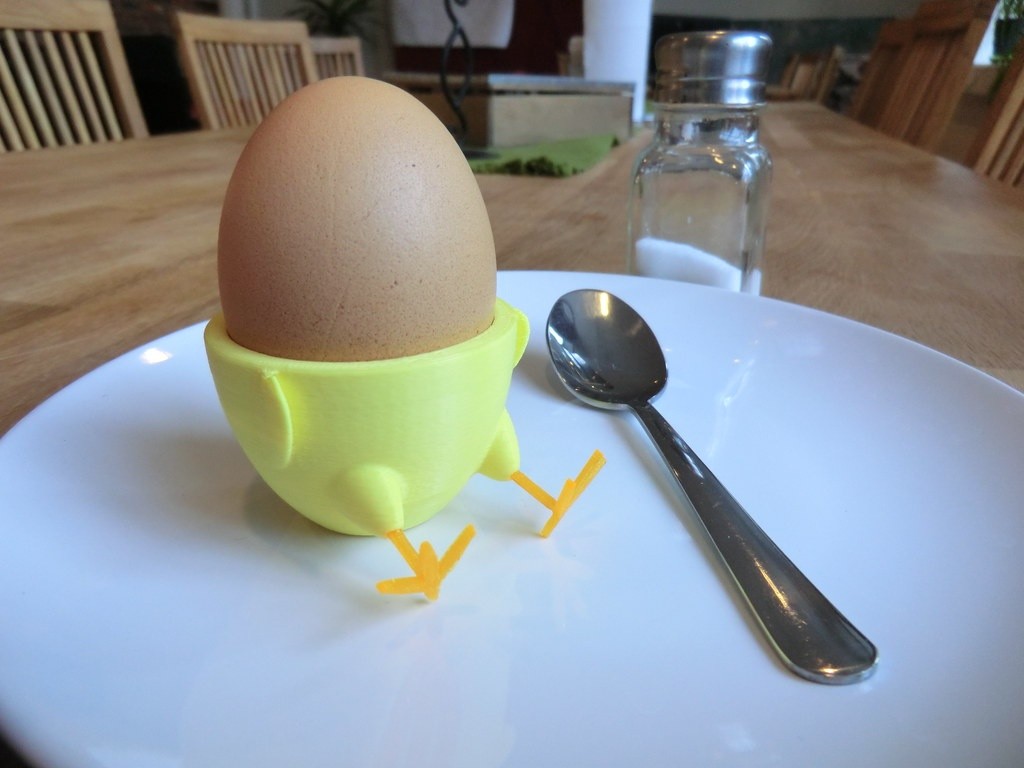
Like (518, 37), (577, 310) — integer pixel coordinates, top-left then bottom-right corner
(555, 54), (567, 76)
(567, 37), (583, 77)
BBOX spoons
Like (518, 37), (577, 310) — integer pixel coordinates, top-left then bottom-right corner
(544, 287), (878, 685)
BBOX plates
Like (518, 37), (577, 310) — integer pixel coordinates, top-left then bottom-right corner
(0, 270), (1024, 768)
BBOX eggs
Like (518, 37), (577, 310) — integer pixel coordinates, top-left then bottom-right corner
(218, 76), (496, 361)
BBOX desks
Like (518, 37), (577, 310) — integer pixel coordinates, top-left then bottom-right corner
(0, 99), (1024, 768)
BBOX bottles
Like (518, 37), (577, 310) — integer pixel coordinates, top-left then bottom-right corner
(621, 25), (780, 299)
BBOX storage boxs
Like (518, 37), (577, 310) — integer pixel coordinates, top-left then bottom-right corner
(383, 72), (637, 149)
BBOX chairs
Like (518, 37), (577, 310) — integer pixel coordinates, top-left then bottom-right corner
(0, 0), (363, 150)
(765, 0), (1024, 189)
